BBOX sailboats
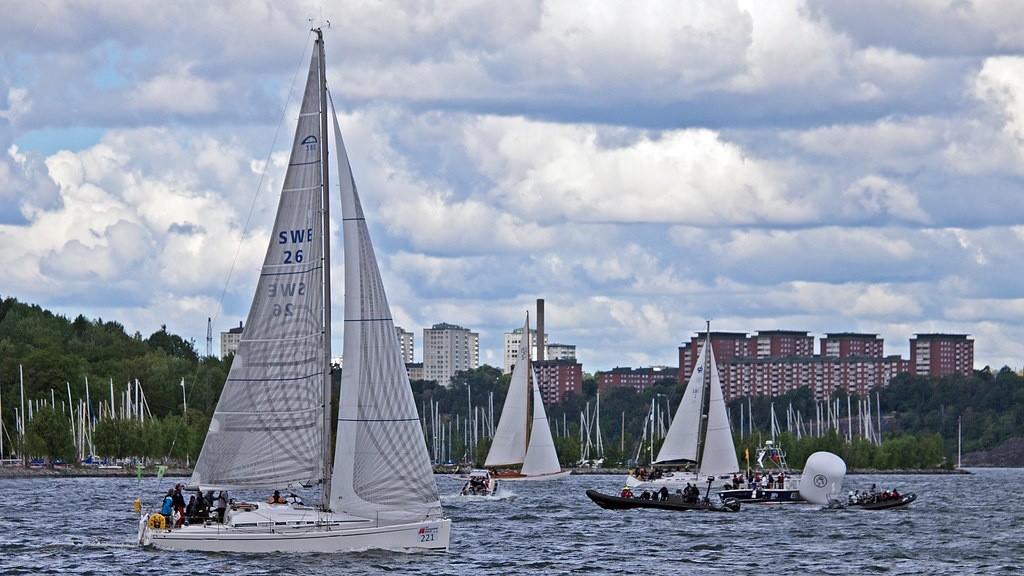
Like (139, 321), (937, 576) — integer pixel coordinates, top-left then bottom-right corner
(412, 383), (479, 476)
(1, 365), (148, 472)
(584, 319), (744, 514)
(483, 309), (575, 483)
(135, 17), (453, 555)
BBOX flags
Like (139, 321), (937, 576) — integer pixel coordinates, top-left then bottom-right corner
(745, 447), (748, 459)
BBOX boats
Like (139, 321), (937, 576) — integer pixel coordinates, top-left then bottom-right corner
(715, 396), (806, 507)
(460, 469), (500, 501)
(824, 490), (918, 512)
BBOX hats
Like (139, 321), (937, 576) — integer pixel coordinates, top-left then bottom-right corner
(624, 487), (630, 489)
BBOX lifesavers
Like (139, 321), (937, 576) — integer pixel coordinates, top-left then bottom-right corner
(149, 514), (165, 529)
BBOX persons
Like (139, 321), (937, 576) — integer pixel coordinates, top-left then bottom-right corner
(746, 466), (784, 490)
(161, 483), (186, 529)
(639, 488), (650, 500)
(620, 486), (635, 498)
(651, 489), (658, 500)
(676, 482), (701, 505)
(268, 490), (284, 504)
(724, 474), (744, 490)
(628, 461), (691, 482)
(491, 467), (498, 477)
(186, 490), (230, 525)
(283, 489), (305, 506)
(658, 483), (669, 501)
(849, 484), (900, 505)
(469, 476), (488, 488)
